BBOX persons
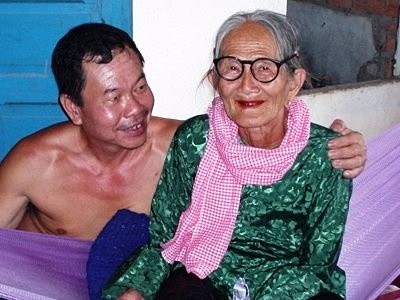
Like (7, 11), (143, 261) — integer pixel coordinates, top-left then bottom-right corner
(0, 22), (367, 300)
(99, 9), (348, 298)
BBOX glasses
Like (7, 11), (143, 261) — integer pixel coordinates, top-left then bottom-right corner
(213, 50), (300, 84)
